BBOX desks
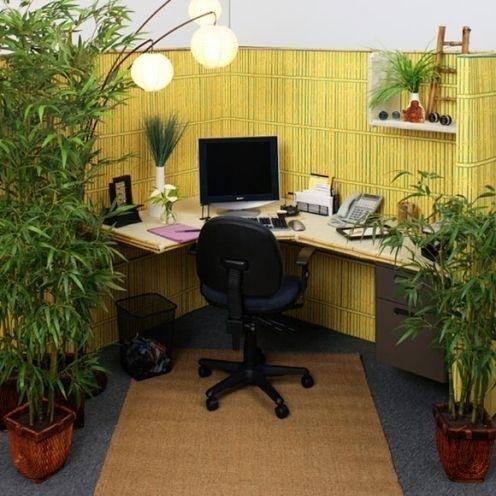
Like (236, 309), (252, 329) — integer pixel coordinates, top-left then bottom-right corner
(80, 198), (451, 278)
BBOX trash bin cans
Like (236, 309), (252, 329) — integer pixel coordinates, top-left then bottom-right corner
(115, 292), (177, 381)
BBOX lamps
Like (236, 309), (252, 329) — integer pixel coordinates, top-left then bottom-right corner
(72, 0), (239, 429)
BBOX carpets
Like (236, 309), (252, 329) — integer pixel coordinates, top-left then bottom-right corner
(93, 347), (404, 496)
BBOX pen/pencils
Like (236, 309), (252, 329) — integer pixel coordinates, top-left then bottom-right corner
(175, 229), (202, 232)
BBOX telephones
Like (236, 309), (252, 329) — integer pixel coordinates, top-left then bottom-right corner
(337, 192), (383, 224)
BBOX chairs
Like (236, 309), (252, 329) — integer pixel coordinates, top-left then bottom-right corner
(196, 216), (316, 419)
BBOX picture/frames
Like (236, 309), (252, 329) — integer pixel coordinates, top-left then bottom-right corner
(337, 225), (393, 240)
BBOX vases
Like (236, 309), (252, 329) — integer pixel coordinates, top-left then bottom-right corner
(160, 202), (177, 225)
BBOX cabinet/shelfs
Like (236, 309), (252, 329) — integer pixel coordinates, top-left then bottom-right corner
(374, 266), (448, 384)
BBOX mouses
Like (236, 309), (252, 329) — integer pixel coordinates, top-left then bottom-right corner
(289, 219), (305, 231)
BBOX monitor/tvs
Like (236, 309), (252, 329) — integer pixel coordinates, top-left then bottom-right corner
(198, 136), (279, 216)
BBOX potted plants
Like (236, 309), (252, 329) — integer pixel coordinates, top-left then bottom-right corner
(0, 0), (150, 484)
(142, 113), (189, 218)
(365, 38), (445, 122)
(345, 169), (496, 484)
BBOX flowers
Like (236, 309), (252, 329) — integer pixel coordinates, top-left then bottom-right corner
(143, 184), (182, 224)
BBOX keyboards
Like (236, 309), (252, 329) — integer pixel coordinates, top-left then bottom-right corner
(206, 217), (288, 231)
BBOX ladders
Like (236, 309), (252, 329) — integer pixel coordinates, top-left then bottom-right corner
(424, 26), (471, 119)
